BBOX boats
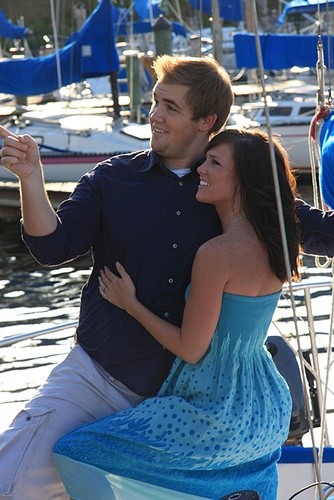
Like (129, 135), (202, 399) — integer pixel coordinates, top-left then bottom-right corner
(1, 113), (153, 183)
(85, 1), (306, 178)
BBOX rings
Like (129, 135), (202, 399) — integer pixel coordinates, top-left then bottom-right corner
(103, 286), (107, 293)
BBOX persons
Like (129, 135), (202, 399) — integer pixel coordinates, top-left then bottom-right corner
(0, 54), (334, 500)
(52, 129), (303, 500)
